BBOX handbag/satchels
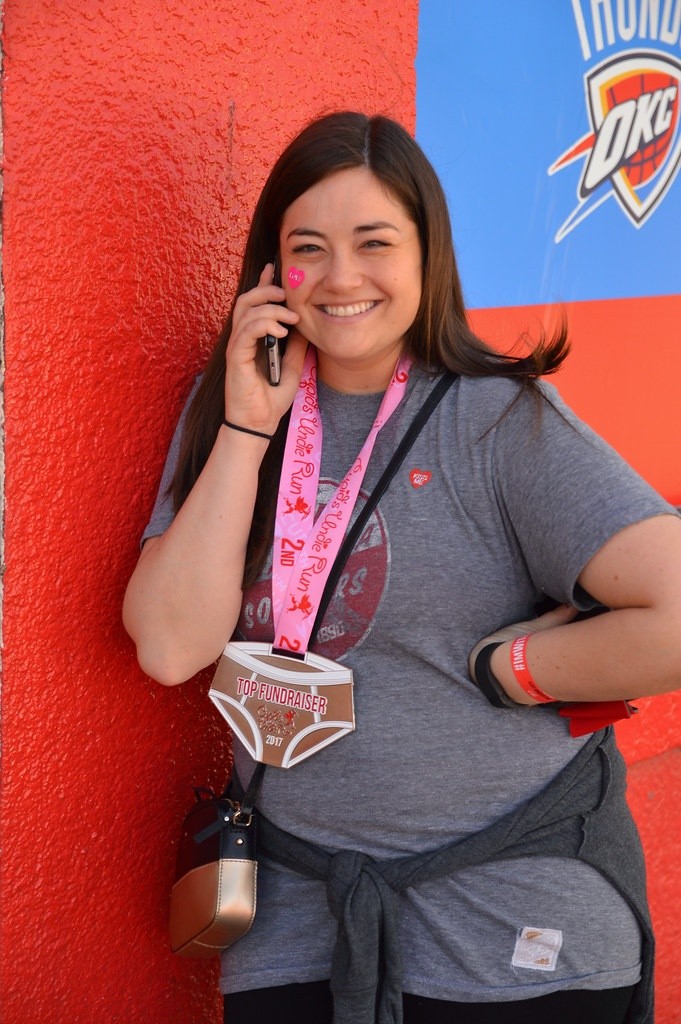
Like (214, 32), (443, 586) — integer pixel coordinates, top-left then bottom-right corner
(166, 783), (259, 959)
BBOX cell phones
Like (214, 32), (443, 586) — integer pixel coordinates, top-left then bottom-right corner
(264, 262), (281, 386)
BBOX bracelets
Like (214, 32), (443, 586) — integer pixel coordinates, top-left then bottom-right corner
(473, 640), (517, 710)
(220, 416), (274, 442)
(508, 632), (558, 703)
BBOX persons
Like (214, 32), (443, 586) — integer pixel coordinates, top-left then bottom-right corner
(120, 109), (681, 1024)
